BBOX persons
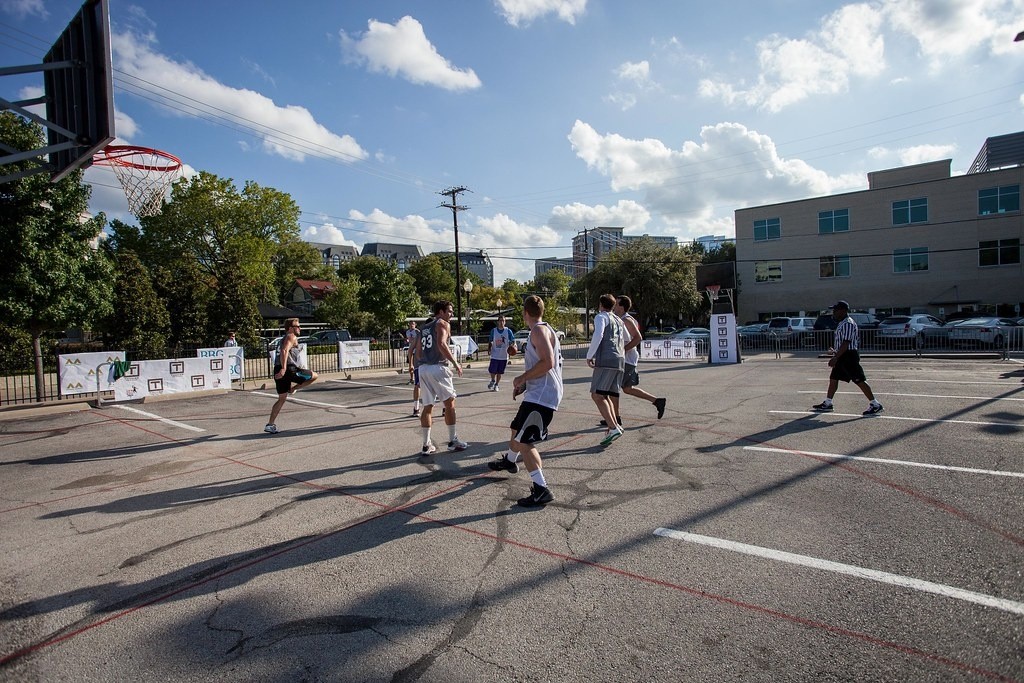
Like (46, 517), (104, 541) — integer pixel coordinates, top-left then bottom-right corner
(417, 300), (468, 455)
(599, 296), (666, 425)
(811, 300), (885, 416)
(487, 315), (515, 392)
(586, 294), (631, 445)
(406, 316), (456, 417)
(488, 296), (563, 506)
(224, 333), (238, 347)
(264, 318), (319, 434)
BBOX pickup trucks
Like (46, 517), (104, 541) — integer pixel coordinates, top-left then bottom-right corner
(297, 330), (377, 346)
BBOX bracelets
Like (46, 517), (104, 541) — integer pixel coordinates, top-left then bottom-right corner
(452, 360), (457, 366)
(521, 383), (526, 390)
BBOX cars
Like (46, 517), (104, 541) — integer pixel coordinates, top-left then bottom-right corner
(513, 328), (566, 353)
(251, 336), (318, 346)
(668, 327), (710, 346)
(736, 312), (1024, 351)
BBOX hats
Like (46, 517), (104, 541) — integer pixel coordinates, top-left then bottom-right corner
(828, 301), (849, 309)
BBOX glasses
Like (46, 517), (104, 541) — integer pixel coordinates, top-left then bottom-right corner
(292, 325), (300, 328)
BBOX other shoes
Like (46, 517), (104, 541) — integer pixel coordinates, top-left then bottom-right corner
(488, 382), (500, 392)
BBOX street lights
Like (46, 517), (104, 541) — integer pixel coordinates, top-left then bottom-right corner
(496, 299), (502, 316)
(463, 279), (473, 336)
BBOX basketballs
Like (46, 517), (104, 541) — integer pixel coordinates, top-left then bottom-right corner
(507, 345), (517, 356)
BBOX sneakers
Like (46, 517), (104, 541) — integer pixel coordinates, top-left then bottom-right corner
(600, 423), (624, 448)
(863, 403), (885, 416)
(518, 482), (555, 506)
(422, 440), (436, 455)
(488, 453), (519, 473)
(406, 379), (415, 385)
(448, 437), (468, 450)
(413, 407), (420, 417)
(264, 423), (281, 434)
(600, 416), (622, 426)
(813, 401), (834, 412)
(442, 408), (456, 416)
(288, 386), (297, 394)
(652, 398), (666, 419)
